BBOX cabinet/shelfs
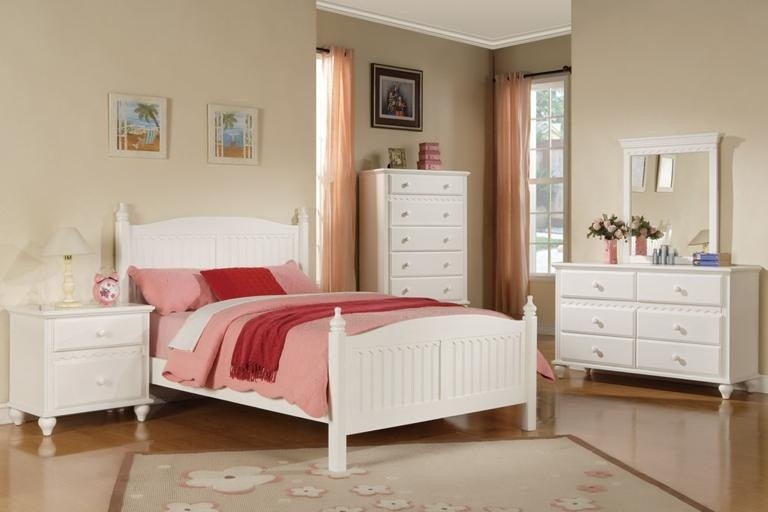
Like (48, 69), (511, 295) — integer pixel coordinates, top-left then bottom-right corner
(357, 168), (471, 305)
(551, 263), (763, 400)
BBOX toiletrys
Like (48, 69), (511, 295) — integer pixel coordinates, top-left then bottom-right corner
(670, 249), (674, 265)
(653, 249), (657, 264)
(661, 246), (666, 264)
(658, 249), (662, 263)
(667, 253), (669, 263)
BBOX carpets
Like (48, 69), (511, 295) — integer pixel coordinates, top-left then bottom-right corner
(107, 435), (715, 512)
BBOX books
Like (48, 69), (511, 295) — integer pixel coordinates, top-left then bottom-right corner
(692, 253), (730, 261)
(692, 261), (730, 267)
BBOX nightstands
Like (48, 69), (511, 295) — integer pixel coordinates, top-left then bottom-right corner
(10, 302), (155, 436)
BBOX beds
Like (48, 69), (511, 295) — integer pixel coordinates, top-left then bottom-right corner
(114, 201), (556, 471)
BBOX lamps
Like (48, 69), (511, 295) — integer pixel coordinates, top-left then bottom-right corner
(40, 227), (94, 308)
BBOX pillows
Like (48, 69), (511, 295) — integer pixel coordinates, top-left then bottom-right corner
(268, 260), (322, 295)
(199, 267), (288, 301)
(128, 267), (214, 315)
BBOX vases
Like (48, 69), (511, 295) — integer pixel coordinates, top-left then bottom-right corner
(635, 237), (647, 255)
(604, 240), (618, 264)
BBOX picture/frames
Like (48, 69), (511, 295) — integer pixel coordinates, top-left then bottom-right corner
(388, 148), (407, 169)
(108, 93), (168, 160)
(208, 104), (259, 165)
(371, 63), (423, 130)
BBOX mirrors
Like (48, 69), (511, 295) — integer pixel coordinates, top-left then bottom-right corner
(617, 132), (720, 266)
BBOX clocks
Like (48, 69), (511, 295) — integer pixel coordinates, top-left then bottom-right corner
(92, 272), (121, 306)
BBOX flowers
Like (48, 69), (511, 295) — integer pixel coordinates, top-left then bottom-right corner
(587, 213), (630, 240)
(632, 214), (664, 240)
